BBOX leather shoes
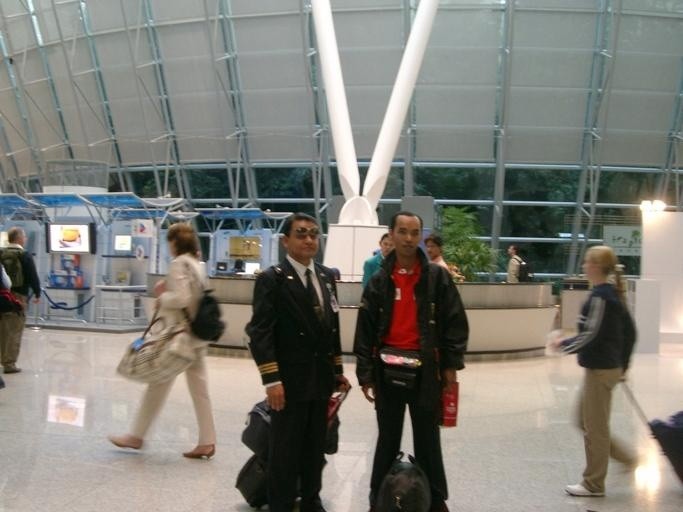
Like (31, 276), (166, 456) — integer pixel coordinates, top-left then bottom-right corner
(4, 364), (22, 374)
(182, 445), (216, 460)
(108, 432), (143, 449)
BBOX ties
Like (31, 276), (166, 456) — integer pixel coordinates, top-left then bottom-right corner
(305, 270), (322, 321)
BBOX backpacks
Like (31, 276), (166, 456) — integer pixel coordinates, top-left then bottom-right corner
(1, 247), (25, 287)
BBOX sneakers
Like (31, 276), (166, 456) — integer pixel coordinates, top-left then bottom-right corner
(564, 482), (605, 497)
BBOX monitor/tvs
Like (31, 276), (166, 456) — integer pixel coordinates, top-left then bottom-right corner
(114, 234), (132, 252)
(46, 394), (86, 427)
(45, 221), (97, 256)
(244, 261), (261, 274)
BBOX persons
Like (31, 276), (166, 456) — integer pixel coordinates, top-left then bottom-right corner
(351, 210), (470, 511)
(423, 235), (449, 272)
(543, 245), (641, 499)
(505, 245), (525, 283)
(244, 210), (351, 511)
(361, 235), (394, 288)
(1, 225), (41, 371)
(111, 224), (217, 459)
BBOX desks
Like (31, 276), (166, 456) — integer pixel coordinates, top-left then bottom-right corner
(43, 285), (148, 327)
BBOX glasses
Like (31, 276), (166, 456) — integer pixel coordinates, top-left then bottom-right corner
(294, 226), (321, 240)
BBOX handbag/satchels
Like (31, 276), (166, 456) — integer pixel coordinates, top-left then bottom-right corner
(241, 400), (274, 460)
(373, 347), (423, 405)
(117, 315), (197, 385)
(176, 259), (225, 343)
(511, 258), (529, 282)
(373, 452), (431, 512)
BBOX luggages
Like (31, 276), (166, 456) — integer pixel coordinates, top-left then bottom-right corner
(620, 377), (683, 480)
(235, 379), (352, 509)
